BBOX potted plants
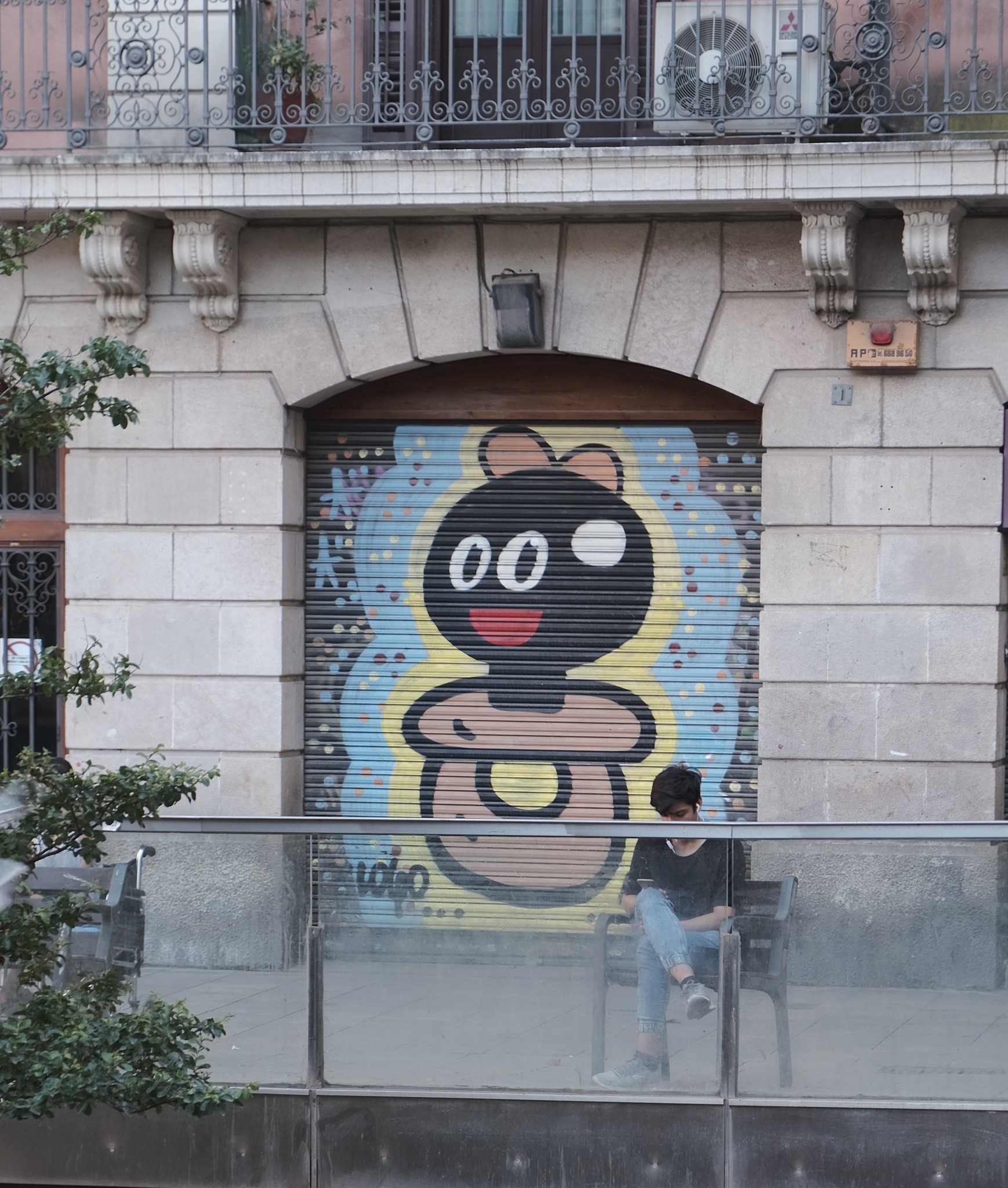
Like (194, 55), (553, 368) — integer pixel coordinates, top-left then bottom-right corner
(255, 31), (321, 143)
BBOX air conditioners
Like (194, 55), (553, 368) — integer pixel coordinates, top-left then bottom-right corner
(653, 0), (834, 136)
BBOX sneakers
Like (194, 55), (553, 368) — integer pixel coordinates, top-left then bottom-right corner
(591, 1057), (662, 1089)
(680, 978), (716, 1020)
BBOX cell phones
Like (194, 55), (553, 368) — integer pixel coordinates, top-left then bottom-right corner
(637, 878), (660, 891)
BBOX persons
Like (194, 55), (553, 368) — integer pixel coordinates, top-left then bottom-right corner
(592, 764), (747, 1092)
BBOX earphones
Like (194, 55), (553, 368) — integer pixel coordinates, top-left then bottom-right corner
(696, 804), (700, 813)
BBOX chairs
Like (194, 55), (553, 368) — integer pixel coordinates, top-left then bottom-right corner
(591, 876), (798, 1087)
